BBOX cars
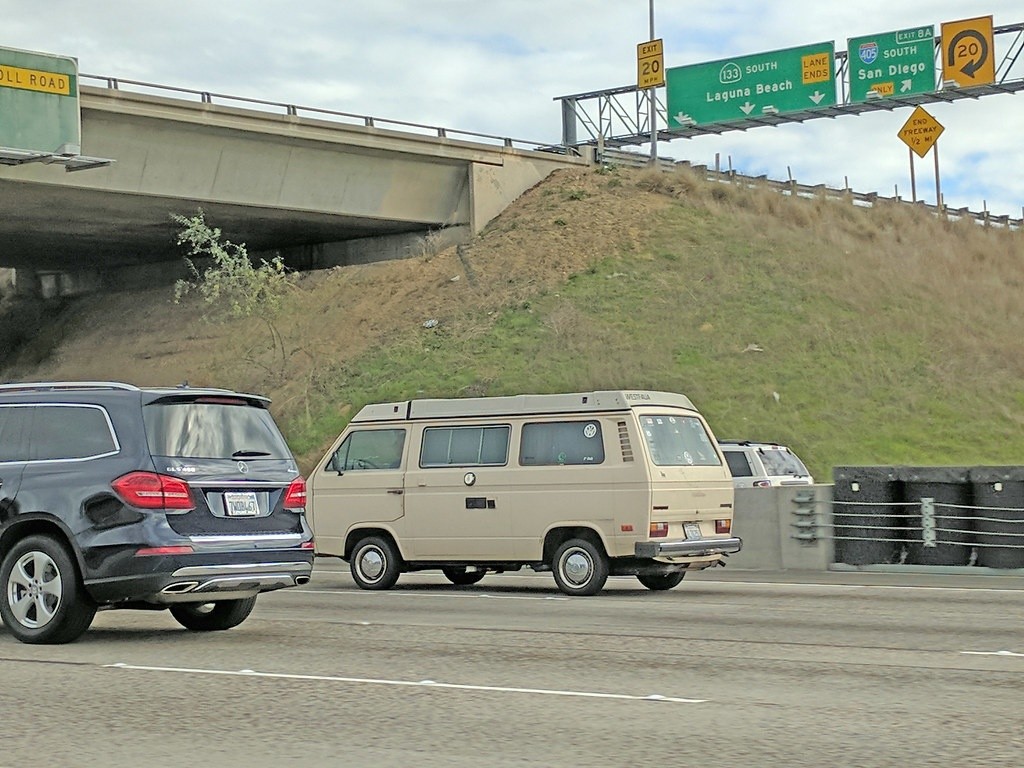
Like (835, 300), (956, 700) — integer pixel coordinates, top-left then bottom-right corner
(715, 436), (815, 489)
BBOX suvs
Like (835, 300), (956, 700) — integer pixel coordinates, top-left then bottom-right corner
(0, 379), (319, 646)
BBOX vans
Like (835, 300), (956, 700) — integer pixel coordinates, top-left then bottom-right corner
(302, 389), (744, 596)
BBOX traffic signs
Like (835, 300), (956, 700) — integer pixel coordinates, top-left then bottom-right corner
(664, 41), (837, 127)
(846, 25), (936, 102)
